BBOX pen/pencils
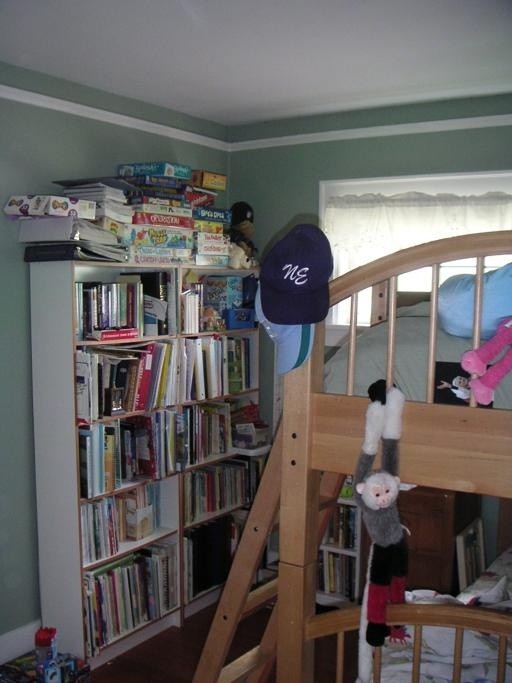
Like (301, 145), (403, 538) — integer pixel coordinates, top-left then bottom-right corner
(35, 626), (56, 647)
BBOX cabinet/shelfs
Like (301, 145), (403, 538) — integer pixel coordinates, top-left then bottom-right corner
(314, 499), (362, 609)
(23, 245), (278, 671)
(363, 485), (479, 598)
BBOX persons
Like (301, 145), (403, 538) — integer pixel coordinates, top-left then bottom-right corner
(435, 374), (494, 403)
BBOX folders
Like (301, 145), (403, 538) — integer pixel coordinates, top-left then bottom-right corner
(16, 216), (128, 263)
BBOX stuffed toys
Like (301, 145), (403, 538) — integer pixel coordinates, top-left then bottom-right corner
(458, 313), (511, 406)
(352, 384), (411, 648)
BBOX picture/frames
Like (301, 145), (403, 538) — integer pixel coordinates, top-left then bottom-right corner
(456, 518), (486, 593)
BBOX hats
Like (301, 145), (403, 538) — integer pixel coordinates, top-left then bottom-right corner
(258, 223), (334, 326)
(254, 280), (317, 377)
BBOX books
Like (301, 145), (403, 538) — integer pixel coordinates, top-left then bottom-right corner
(3, 170), (138, 262)
(77, 266), (181, 661)
(181, 283), (203, 335)
(166, 402), (271, 477)
(183, 504), (252, 604)
(318, 474), (357, 601)
(180, 335), (251, 402)
(180, 456), (265, 530)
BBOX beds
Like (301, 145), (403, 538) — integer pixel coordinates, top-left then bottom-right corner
(278, 229), (512, 683)
